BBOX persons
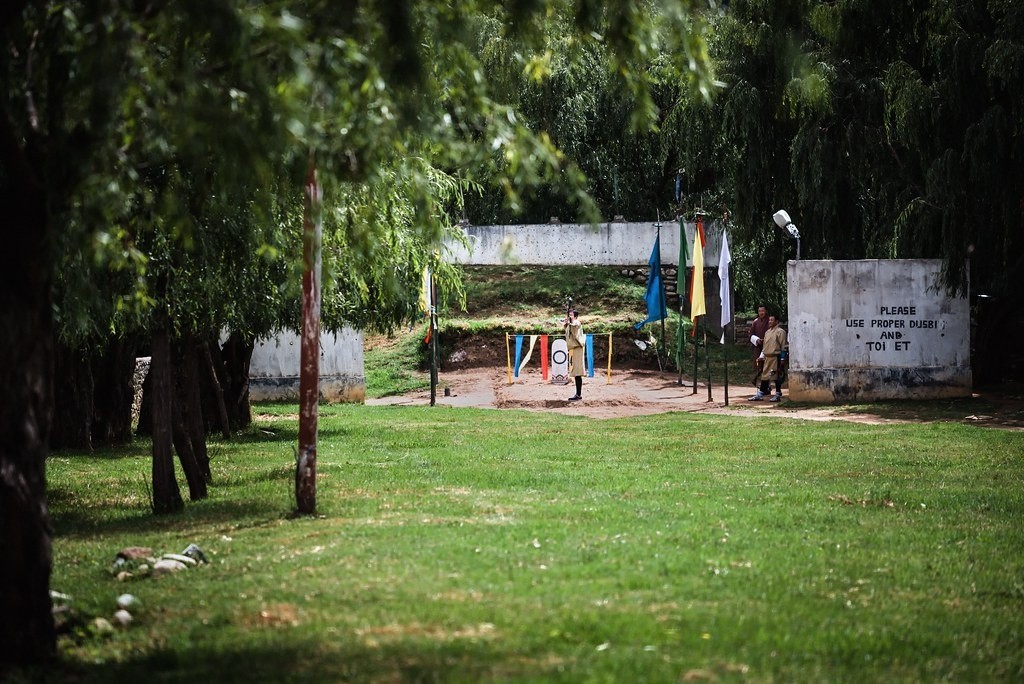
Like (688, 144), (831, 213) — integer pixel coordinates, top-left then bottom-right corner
(748, 304), (772, 395)
(563, 308), (585, 401)
(747, 314), (787, 402)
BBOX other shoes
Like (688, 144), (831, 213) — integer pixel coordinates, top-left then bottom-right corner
(568, 395), (582, 401)
(770, 396), (780, 402)
(748, 396), (763, 401)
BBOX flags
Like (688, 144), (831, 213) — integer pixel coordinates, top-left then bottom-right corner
(421, 265), (433, 320)
(632, 229), (668, 327)
(676, 216), (689, 296)
(718, 227), (733, 331)
(686, 217), (707, 337)
